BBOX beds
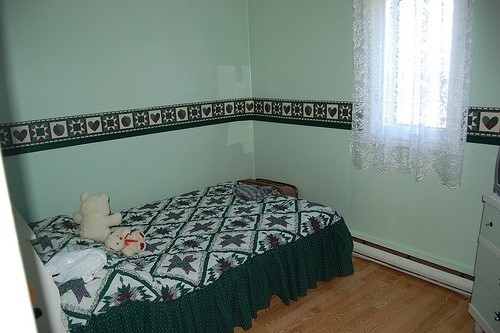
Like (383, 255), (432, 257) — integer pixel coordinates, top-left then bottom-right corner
(25, 179), (355, 333)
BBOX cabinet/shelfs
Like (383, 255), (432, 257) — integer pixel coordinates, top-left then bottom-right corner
(465, 193), (500, 333)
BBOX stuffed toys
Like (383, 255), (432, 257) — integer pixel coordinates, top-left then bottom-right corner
(104, 230), (147, 257)
(72, 192), (124, 243)
